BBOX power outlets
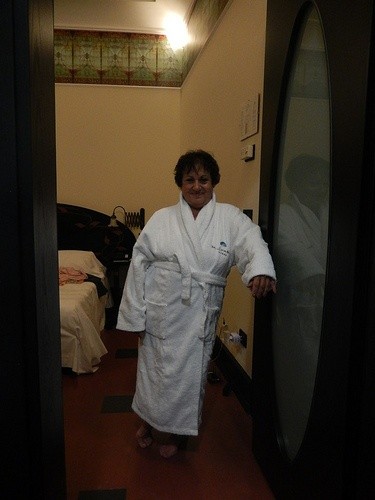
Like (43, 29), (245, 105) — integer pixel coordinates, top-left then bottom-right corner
(239, 328), (247, 348)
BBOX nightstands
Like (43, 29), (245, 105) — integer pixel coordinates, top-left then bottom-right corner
(111, 260), (132, 317)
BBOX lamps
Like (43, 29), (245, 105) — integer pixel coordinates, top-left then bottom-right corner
(107, 205), (126, 228)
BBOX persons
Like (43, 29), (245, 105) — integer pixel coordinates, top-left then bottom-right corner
(116, 149), (277, 458)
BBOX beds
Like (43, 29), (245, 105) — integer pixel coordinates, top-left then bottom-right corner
(58, 202), (136, 373)
